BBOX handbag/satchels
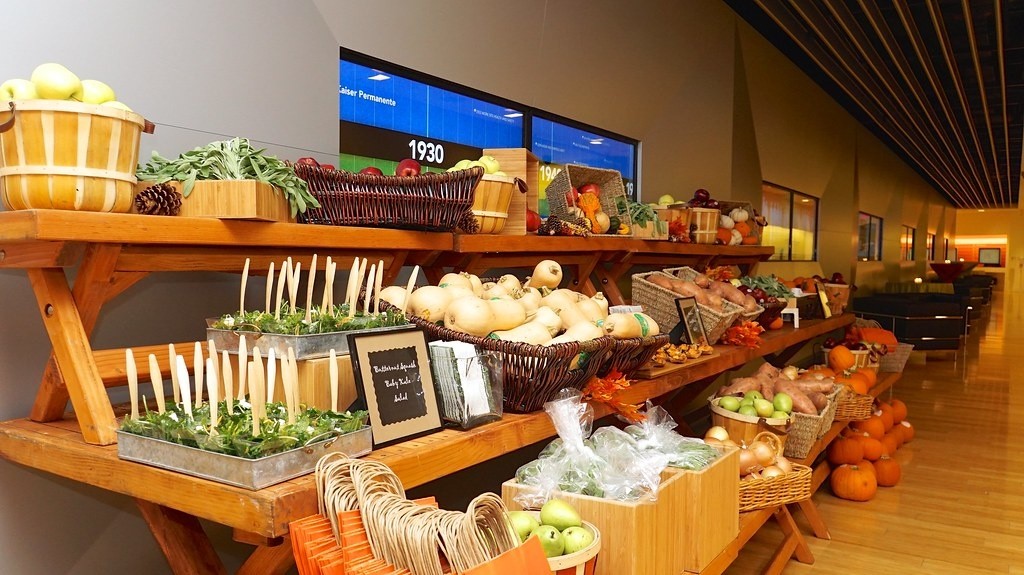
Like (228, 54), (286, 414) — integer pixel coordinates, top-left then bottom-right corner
(288, 452), (551, 575)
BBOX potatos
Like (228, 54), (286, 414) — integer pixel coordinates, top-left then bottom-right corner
(719, 363), (835, 416)
(646, 272), (758, 313)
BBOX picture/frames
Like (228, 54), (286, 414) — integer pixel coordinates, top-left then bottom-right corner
(668, 295), (711, 349)
(346, 324), (446, 451)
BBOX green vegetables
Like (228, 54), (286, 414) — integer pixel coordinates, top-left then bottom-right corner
(136, 135), (321, 218)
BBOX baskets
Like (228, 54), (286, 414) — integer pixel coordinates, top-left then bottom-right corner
(718, 200), (760, 241)
(739, 461), (811, 513)
(784, 400), (831, 459)
(283, 161), (484, 231)
(826, 384), (844, 424)
(478, 277), (669, 381)
(881, 342), (914, 374)
(632, 270), (743, 346)
(834, 393), (873, 422)
(545, 163), (635, 238)
(359, 284), (614, 413)
(663, 265), (766, 326)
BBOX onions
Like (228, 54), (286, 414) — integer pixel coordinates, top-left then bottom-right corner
(702, 425), (793, 480)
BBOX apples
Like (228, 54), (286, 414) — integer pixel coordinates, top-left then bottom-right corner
(296, 154), (514, 183)
(647, 188), (717, 208)
(0, 63), (133, 114)
(738, 285), (779, 306)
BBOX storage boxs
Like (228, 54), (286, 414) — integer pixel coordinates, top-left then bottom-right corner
(0, 98), (155, 213)
(131, 178), (299, 225)
(739, 430), (813, 512)
(470, 172), (517, 235)
(630, 271), (744, 347)
(282, 159), (485, 232)
(781, 338), (913, 460)
(711, 396), (794, 456)
(604, 333), (671, 383)
(501, 465), (685, 575)
(544, 163), (637, 238)
(482, 146), (540, 235)
(752, 279), (852, 330)
(363, 277), (615, 415)
(663, 267), (765, 328)
(632, 196), (761, 245)
(668, 442), (741, 573)
(505, 510), (602, 575)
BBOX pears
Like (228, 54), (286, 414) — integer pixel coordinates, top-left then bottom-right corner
(719, 389), (793, 418)
(507, 501), (593, 557)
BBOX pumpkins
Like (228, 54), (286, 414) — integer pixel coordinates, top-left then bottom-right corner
(716, 207), (763, 246)
(800, 343), (917, 501)
(377, 261), (659, 345)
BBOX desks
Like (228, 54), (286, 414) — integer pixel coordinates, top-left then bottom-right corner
(423, 237), (635, 313)
(592, 241), (775, 307)
(0, 218), (452, 447)
